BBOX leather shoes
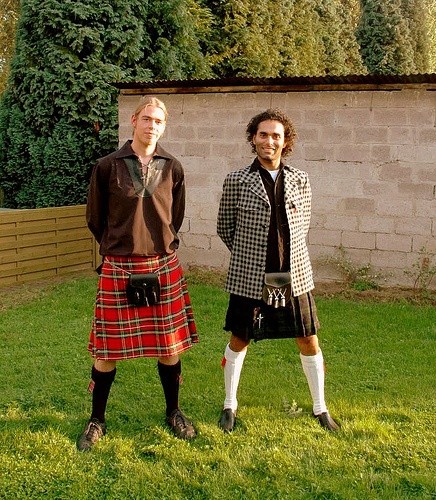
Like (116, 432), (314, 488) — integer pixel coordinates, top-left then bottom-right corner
(218, 407), (238, 434)
(312, 411), (338, 430)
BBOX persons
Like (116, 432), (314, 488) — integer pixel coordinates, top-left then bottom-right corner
(215, 110), (342, 436)
(76, 96), (197, 454)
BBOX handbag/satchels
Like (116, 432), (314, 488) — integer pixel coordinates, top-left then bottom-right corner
(262, 272), (292, 309)
(126, 273), (160, 307)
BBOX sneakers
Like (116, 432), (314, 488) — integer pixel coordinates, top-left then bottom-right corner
(166, 409), (196, 439)
(76, 418), (107, 451)
